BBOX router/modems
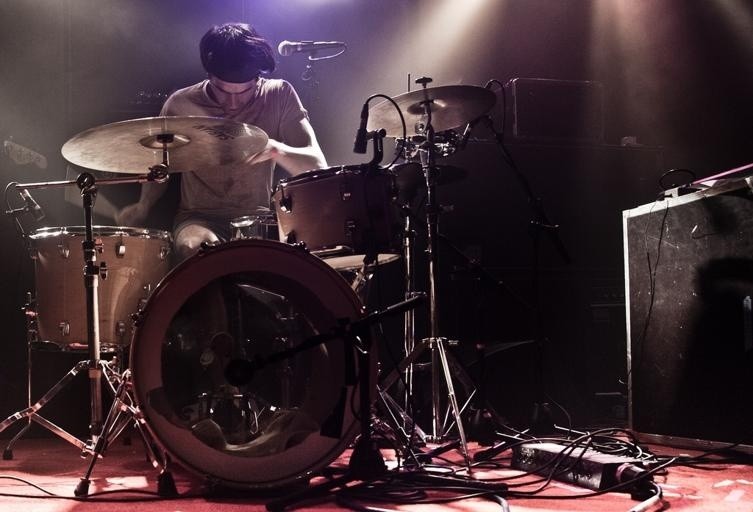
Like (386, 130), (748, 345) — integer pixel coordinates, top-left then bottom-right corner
(511, 440), (653, 495)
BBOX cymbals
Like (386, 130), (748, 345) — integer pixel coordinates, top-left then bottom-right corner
(366, 85), (497, 137)
(61, 115), (268, 173)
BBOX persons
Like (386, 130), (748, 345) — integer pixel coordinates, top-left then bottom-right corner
(115, 23), (330, 444)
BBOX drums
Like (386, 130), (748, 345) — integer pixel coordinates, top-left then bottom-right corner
(274, 165), (404, 271)
(129, 238), (383, 490)
(27, 225), (172, 353)
(229, 214), (281, 240)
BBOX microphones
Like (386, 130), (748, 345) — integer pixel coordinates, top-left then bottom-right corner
(14, 182), (45, 223)
(353, 103), (373, 155)
(221, 294), (424, 386)
(279, 40), (346, 58)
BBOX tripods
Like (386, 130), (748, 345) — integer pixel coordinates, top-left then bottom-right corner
(0, 187), (177, 500)
(377, 142), (508, 478)
(264, 327), (510, 511)
